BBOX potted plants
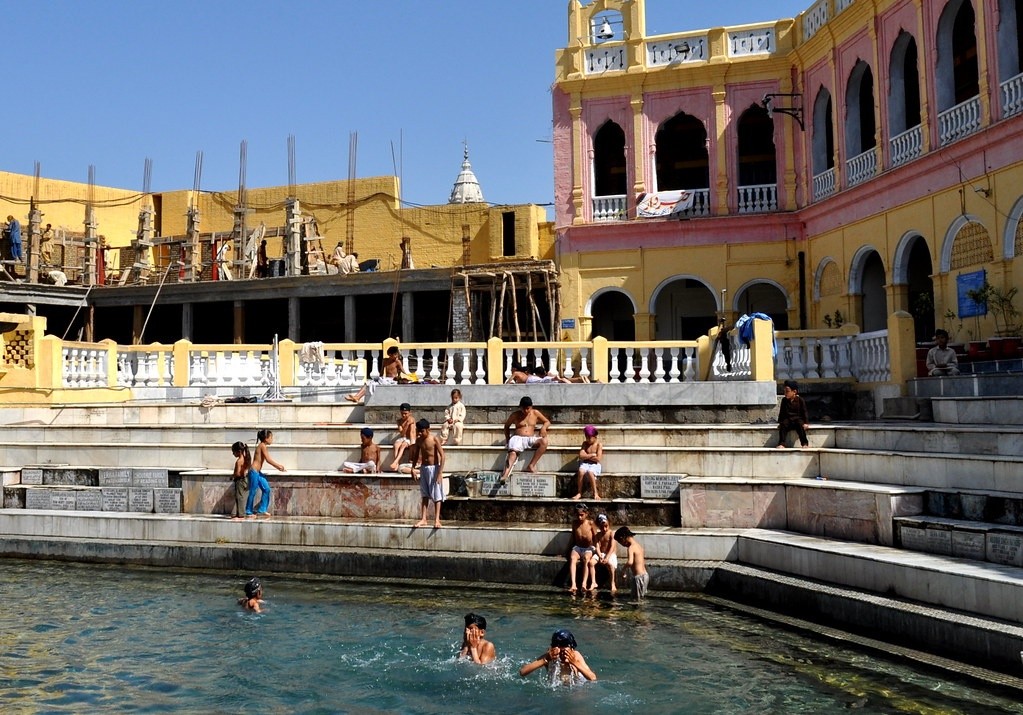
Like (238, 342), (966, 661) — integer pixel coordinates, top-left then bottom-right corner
(914, 286), (1023, 357)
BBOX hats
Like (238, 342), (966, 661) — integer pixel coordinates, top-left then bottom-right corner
(245, 578), (262, 599)
(552, 629), (578, 650)
(595, 514), (607, 525)
(584, 426), (598, 436)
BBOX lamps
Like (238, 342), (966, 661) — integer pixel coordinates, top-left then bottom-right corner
(597, 16), (613, 39)
(675, 41), (690, 53)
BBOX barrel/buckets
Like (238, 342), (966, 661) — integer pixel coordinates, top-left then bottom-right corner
(464, 466), (484, 498)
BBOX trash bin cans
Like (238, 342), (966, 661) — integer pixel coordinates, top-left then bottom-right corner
(269, 260), (285, 277)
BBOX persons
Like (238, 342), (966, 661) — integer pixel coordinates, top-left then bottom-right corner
(41, 224), (54, 265)
(569, 502), (649, 602)
(343, 389), (466, 528)
(926, 329), (960, 376)
(231, 441), (251, 518)
(458, 613), (495, 666)
(776, 380), (809, 449)
(237, 578), (264, 614)
(572, 426), (602, 501)
(500, 396), (550, 480)
(331, 241), (359, 274)
(519, 629), (596, 683)
(244, 429), (286, 518)
(5, 215), (25, 263)
(506, 361), (602, 383)
(218, 244), (231, 280)
(346, 345), (408, 403)
(256, 240), (268, 278)
(43, 270), (68, 286)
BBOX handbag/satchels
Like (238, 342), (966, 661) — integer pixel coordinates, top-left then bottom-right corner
(449, 472), (468, 497)
(466, 469), (485, 498)
